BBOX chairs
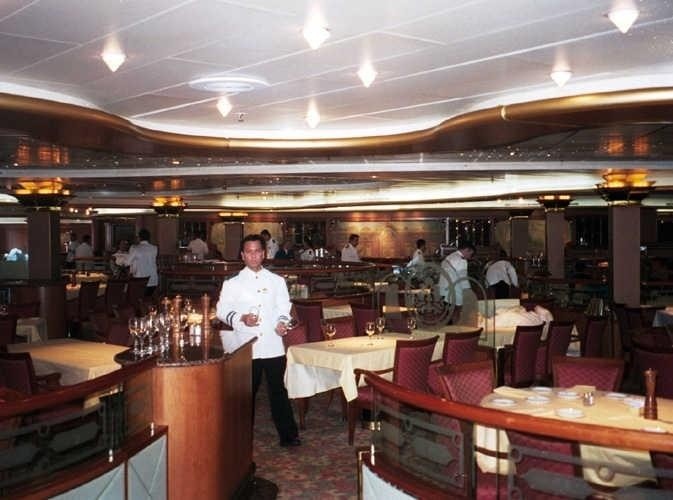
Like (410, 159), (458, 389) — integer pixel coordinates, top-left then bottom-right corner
(0, 352), (107, 475)
(65, 276), (149, 320)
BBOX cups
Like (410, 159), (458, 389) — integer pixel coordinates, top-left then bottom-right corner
(280, 318), (297, 332)
(248, 306), (259, 321)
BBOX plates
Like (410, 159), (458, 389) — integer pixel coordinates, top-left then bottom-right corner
(488, 386), (667, 433)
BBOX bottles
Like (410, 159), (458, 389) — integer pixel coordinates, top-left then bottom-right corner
(160, 295), (183, 327)
(188, 322), (200, 335)
(200, 294), (210, 329)
(71, 272), (76, 287)
(171, 330), (211, 362)
(642, 369), (658, 419)
(289, 283), (308, 298)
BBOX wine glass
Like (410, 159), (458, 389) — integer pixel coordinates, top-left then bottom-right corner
(365, 322), (375, 345)
(128, 315), (171, 357)
(375, 318), (384, 340)
(407, 318), (416, 341)
(319, 318), (336, 348)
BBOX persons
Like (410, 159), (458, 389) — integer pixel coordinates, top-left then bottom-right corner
(186, 232), (209, 262)
(407, 238), (427, 267)
(110, 229), (159, 298)
(440, 241), (520, 325)
(216, 234), (301, 446)
(65, 231), (95, 270)
(341, 234), (363, 263)
(261, 229), (296, 259)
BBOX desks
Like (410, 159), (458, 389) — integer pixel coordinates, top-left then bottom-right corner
(7, 338), (132, 399)
